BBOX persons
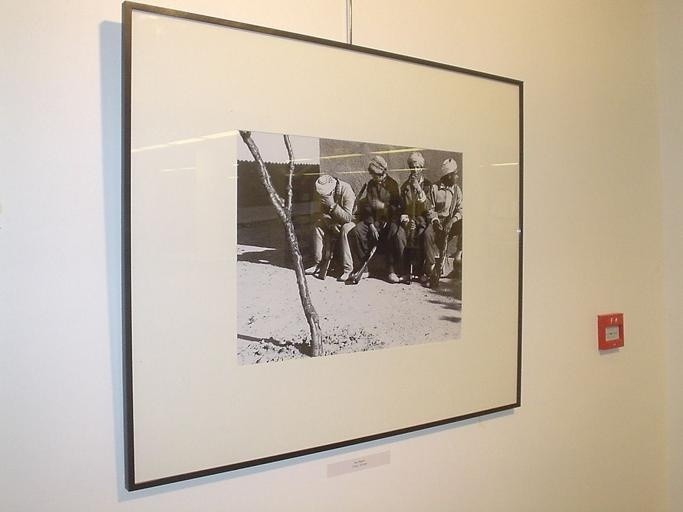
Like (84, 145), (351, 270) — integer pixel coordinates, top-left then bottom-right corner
(345, 155), (400, 284)
(395, 150), (434, 288)
(419, 156), (461, 290)
(301, 175), (359, 282)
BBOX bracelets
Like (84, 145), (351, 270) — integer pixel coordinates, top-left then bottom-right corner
(454, 215), (459, 222)
(417, 189), (421, 194)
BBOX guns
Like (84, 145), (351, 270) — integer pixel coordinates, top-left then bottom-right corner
(430, 187), (459, 286)
(318, 231), (338, 280)
(408, 189), (416, 285)
(353, 220), (388, 284)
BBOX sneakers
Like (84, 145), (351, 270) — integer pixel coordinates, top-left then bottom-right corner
(304, 263), (441, 288)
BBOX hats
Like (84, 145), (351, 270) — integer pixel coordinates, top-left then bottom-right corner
(369, 155), (387, 173)
(440, 158), (457, 179)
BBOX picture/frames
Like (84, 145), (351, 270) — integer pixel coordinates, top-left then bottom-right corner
(120, 0), (524, 493)
